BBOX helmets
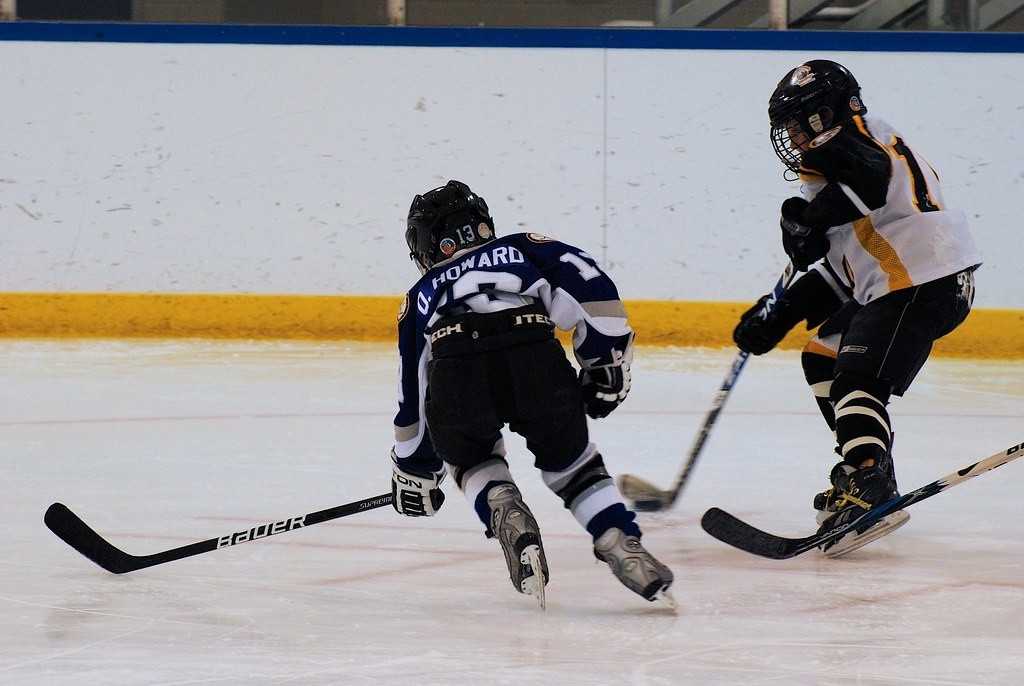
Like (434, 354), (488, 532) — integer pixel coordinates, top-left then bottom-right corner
(405, 180), (496, 260)
(767, 59), (867, 140)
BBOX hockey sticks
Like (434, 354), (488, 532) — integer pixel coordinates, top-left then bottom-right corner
(40, 488), (410, 574)
(616, 250), (800, 511)
(700, 438), (1023, 560)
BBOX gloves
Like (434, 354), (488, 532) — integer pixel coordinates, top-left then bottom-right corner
(574, 348), (633, 419)
(733, 293), (804, 356)
(778, 197), (830, 273)
(390, 445), (449, 517)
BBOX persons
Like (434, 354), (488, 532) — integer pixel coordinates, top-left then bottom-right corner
(391, 180), (679, 611)
(732, 60), (983, 557)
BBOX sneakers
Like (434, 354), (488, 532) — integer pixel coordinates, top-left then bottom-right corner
(473, 479), (550, 611)
(586, 503), (679, 613)
(814, 431), (910, 556)
